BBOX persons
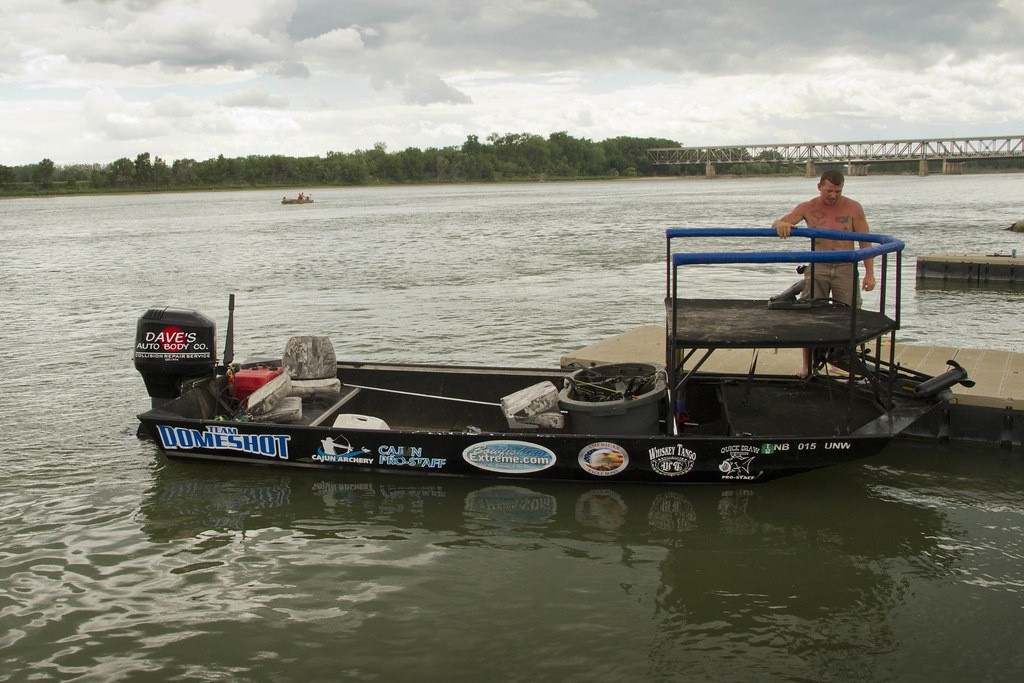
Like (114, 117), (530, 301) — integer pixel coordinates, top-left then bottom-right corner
(772, 170), (875, 378)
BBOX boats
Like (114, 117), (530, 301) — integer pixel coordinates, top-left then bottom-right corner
(132, 228), (977, 485)
(280, 194), (314, 204)
